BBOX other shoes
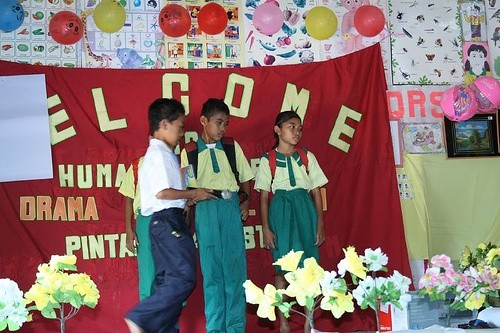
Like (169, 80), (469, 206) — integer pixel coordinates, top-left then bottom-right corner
(124, 318), (144, 333)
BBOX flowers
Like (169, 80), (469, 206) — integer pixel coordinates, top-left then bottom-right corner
(0, 277), (33, 332)
(322, 247), (411, 333)
(416, 242), (500, 327)
(25, 254), (100, 333)
(242, 246), (366, 333)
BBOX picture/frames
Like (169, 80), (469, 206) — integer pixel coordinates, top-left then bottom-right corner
(444, 113), (499, 158)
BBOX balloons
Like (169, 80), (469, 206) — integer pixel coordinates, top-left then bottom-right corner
(158, 4), (191, 37)
(93, 0), (126, 33)
(0, 0), (24, 31)
(354, 5), (385, 37)
(252, 3), (283, 36)
(198, 3), (228, 35)
(49, 11), (83, 44)
(306, 6), (338, 40)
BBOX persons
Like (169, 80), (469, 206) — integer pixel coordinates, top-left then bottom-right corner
(254, 111), (329, 333)
(116, 133), (157, 303)
(123, 98), (218, 333)
(181, 98), (255, 333)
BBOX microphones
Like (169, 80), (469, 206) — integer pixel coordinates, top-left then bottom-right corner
(211, 189), (232, 200)
